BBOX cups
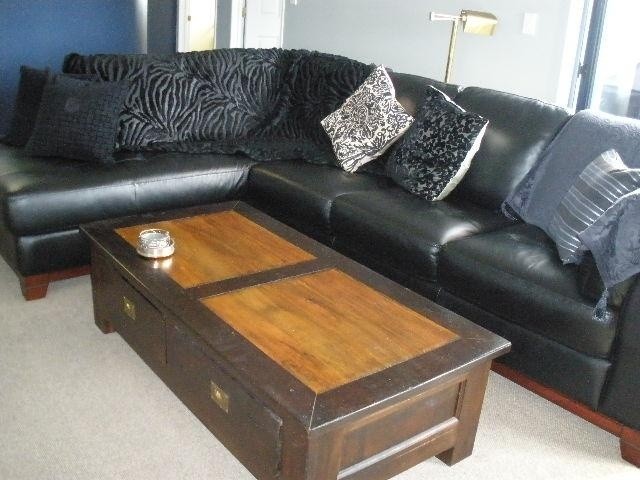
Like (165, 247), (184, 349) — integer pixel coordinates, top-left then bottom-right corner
(138, 228), (171, 252)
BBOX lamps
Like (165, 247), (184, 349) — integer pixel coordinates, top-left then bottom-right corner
(428, 10), (498, 84)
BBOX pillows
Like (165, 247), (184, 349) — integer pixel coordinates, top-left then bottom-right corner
(319, 64), (414, 175)
(2, 66), (102, 147)
(385, 84), (489, 202)
(1, 48), (639, 466)
(22, 67), (133, 166)
(502, 118), (640, 266)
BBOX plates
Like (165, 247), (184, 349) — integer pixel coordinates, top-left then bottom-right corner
(136, 245), (175, 259)
